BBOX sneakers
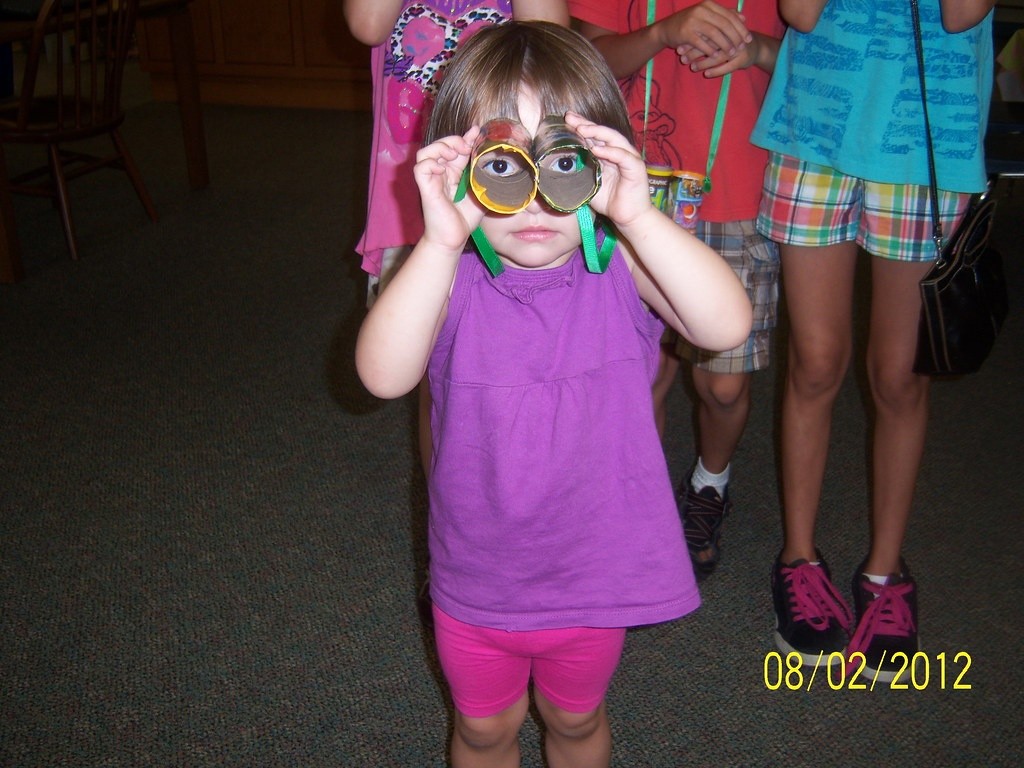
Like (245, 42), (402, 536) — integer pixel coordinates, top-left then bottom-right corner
(681, 462), (734, 583)
(769, 547), (855, 667)
(852, 556), (920, 682)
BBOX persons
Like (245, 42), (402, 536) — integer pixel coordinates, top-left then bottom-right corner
(355, 19), (753, 768)
(568, 0), (787, 577)
(345, 0), (571, 488)
(749, 0), (995, 683)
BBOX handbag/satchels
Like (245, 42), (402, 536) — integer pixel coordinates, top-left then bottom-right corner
(919, 172), (1009, 379)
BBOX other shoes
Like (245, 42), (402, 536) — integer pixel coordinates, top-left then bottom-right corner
(329, 305), (387, 415)
